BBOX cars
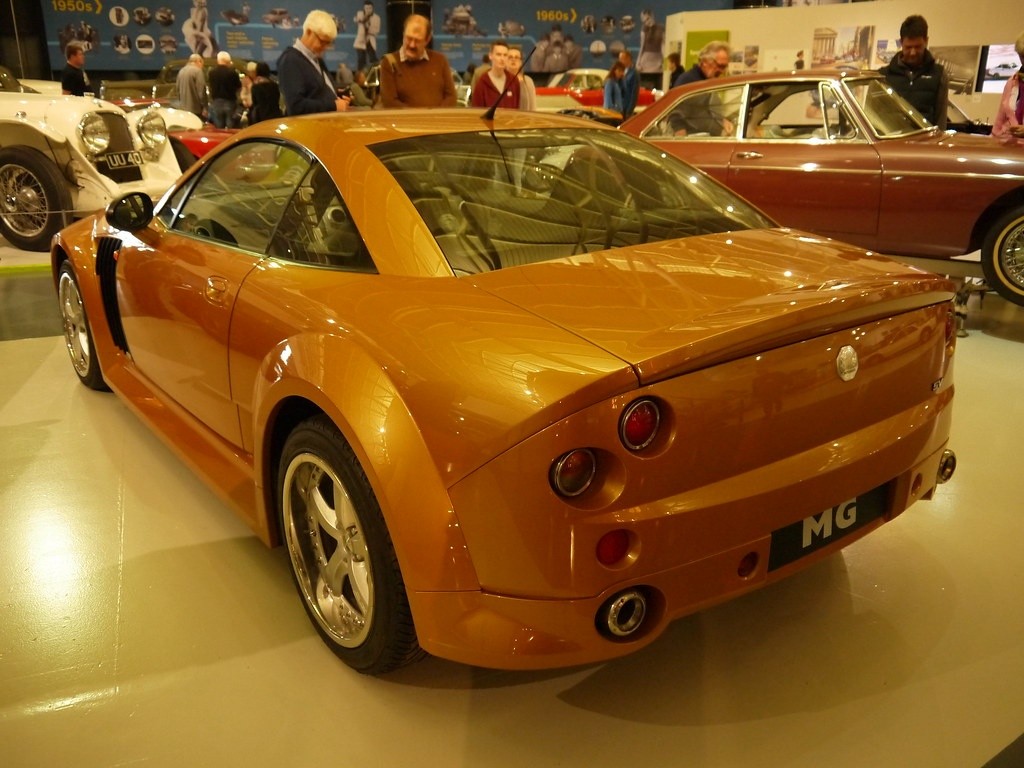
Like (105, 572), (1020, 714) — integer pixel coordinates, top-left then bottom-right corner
(0, 70), (667, 253)
(617, 71), (1024, 307)
(50, 107), (957, 674)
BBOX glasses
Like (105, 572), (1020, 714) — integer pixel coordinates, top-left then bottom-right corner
(508, 56), (522, 63)
(313, 31), (331, 48)
(713, 58), (727, 70)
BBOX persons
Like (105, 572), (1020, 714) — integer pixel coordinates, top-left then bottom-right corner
(602, 52), (638, 119)
(860, 14), (948, 135)
(585, 16), (596, 32)
(667, 53), (685, 89)
(241, 61), (283, 123)
(378, 15), (456, 107)
(276, 10), (355, 115)
(181, 0), (218, 58)
(64, 20), (94, 50)
(462, 40), (537, 193)
(207, 51), (240, 129)
(336, 63), (353, 85)
(112, 7), (176, 54)
(670, 39), (732, 137)
(353, 2), (381, 70)
(991, 30), (1024, 138)
(60, 44), (93, 97)
(635, 9), (664, 89)
(176, 54), (206, 116)
(530, 22), (585, 72)
